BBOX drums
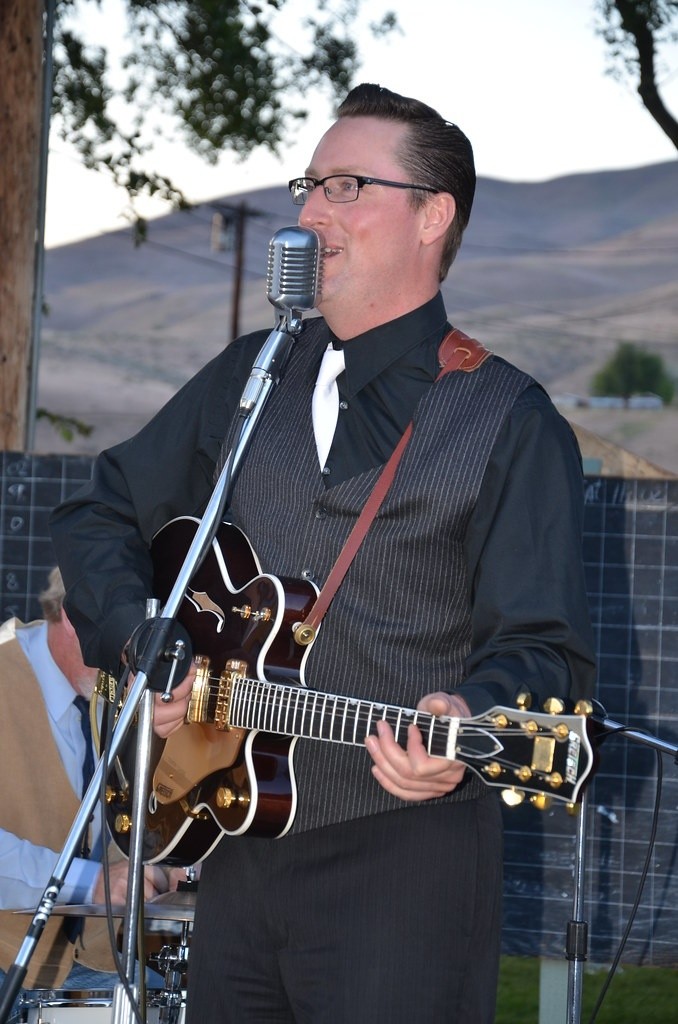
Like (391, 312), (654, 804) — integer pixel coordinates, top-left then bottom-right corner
(21, 986), (182, 1024)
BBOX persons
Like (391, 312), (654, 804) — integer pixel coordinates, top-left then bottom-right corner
(50, 84), (595, 1024)
(0, 568), (202, 1024)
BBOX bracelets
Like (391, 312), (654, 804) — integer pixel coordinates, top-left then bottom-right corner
(183, 866), (196, 883)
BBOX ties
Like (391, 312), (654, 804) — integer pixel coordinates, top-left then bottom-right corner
(72, 696), (101, 802)
(311, 340), (346, 471)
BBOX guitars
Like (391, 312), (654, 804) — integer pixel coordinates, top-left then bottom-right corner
(96, 516), (605, 871)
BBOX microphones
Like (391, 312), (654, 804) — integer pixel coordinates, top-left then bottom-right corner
(241, 225), (327, 419)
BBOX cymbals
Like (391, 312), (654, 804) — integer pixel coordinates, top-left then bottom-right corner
(12, 890), (196, 924)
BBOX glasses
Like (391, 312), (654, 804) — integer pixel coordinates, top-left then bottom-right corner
(289, 171), (444, 206)
